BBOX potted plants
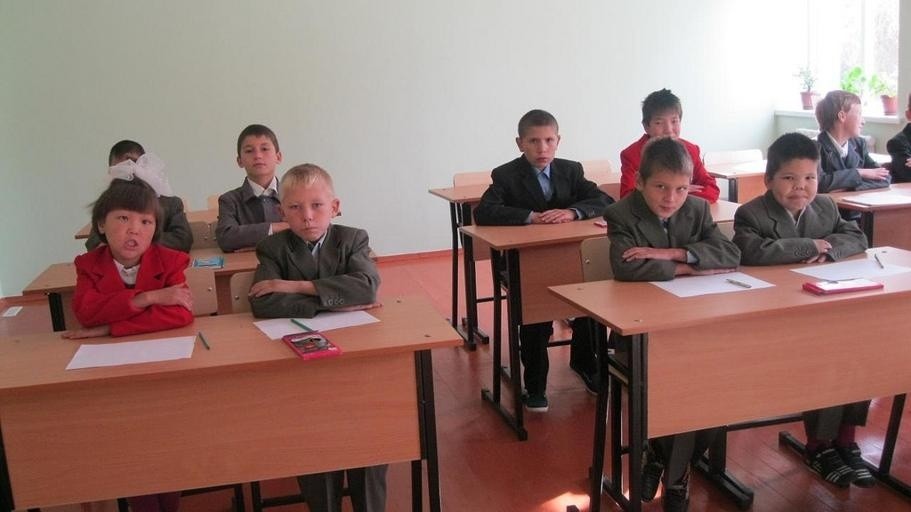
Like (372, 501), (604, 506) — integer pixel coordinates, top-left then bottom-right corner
(867, 75), (899, 117)
(800, 78), (820, 112)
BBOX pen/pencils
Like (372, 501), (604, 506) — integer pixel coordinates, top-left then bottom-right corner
(726, 279), (751, 287)
(874, 254), (884, 268)
(290, 319), (314, 332)
(198, 332), (210, 350)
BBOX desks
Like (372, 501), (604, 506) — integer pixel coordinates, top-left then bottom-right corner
(431, 151), (911, 511)
(2, 193), (464, 512)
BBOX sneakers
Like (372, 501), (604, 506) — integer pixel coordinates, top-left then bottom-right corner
(660, 464), (692, 512)
(640, 442), (664, 504)
(840, 442), (876, 488)
(577, 362), (607, 395)
(799, 446), (856, 485)
(525, 389), (549, 413)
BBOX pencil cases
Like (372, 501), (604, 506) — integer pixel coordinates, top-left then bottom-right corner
(802, 278), (884, 295)
(282, 331), (342, 361)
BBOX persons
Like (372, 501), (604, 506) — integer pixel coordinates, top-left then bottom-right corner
(886, 90), (911, 182)
(473, 110), (617, 415)
(64, 181), (194, 510)
(85, 140), (194, 252)
(732, 133), (879, 490)
(604, 132), (741, 511)
(807, 91), (892, 192)
(250, 164), (388, 511)
(214, 124), (295, 255)
(619, 90), (719, 204)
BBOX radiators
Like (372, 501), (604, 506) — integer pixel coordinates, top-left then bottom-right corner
(795, 127), (877, 153)
